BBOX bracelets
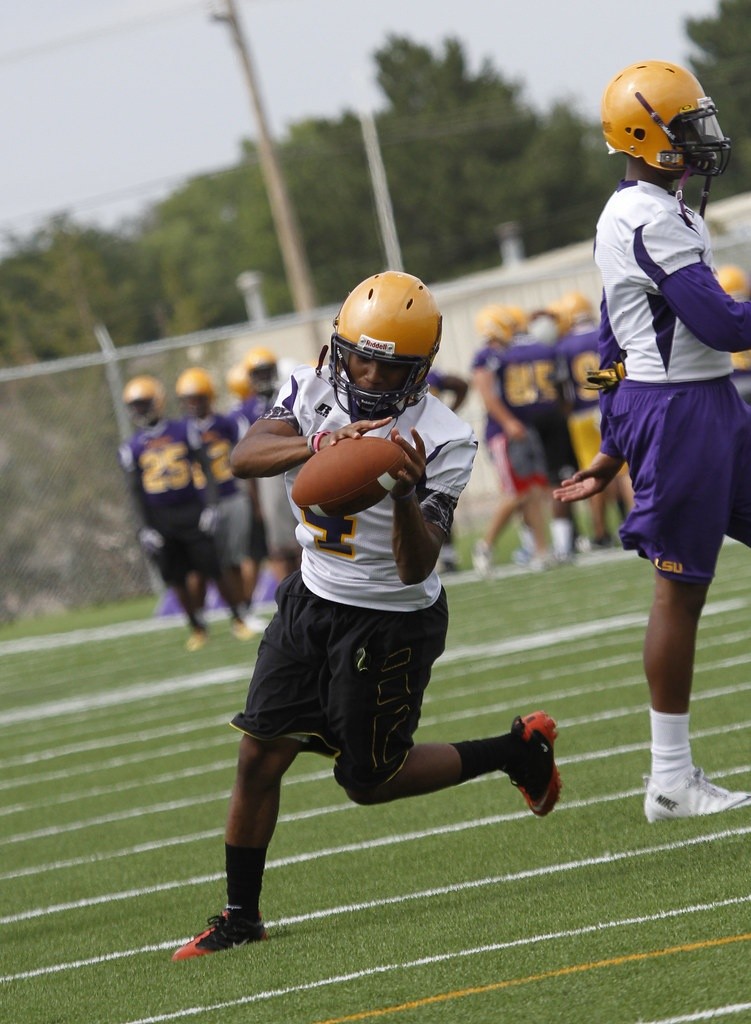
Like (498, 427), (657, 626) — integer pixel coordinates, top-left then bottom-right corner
(389, 485), (416, 500)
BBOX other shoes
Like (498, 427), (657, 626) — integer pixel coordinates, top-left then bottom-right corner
(470, 539), (495, 581)
(530, 552), (574, 573)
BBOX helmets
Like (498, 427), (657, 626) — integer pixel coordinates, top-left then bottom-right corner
(174, 367), (215, 415)
(122, 376), (166, 428)
(477, 305), (529, 350)
(225, 364), (251, 399)
(603, 62), (732, 176)
(241, 347), (280, 394)
(548, 291), (593, 338)
(713, 262), (750, 301)
(316, 270), (442, 423)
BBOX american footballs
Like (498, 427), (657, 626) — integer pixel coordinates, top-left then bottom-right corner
(290, 433), (407, 519)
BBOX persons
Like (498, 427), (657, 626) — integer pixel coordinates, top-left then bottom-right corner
(552, 60), (751, 821)
(114, 264), (751, 655)
(167, 271), (563, 964)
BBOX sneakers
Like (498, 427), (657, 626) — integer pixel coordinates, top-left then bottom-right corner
(509, 710), (563, 816)
(172, 911), (267, 960)
(186, 623), (207, 651)
(228, 616), (253, 642)
(642, 765), (750, 823)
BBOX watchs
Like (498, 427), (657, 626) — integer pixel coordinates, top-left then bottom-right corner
(308, 429), (332, 455)
